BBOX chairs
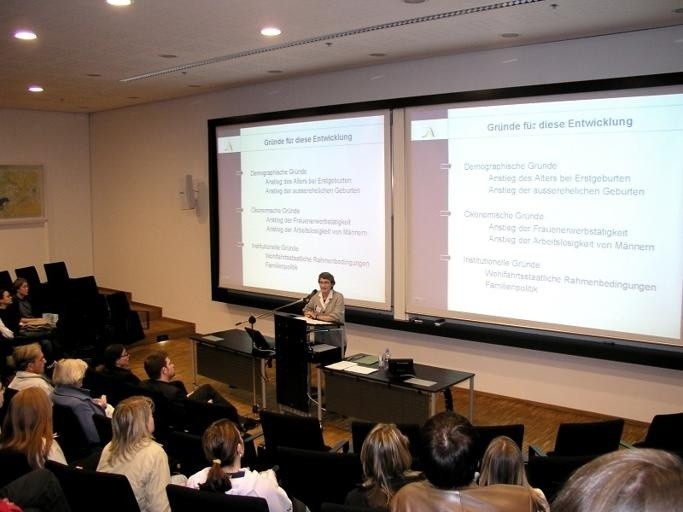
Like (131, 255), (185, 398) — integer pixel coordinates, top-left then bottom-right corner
(0, 260), (682, 511)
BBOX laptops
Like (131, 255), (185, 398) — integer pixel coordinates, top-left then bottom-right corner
(245, 327), (275, 349)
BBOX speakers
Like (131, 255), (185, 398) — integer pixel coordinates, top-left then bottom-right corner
(177, 174), (197, 210)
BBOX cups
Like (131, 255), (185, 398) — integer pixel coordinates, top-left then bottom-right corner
(48, 314), (58, 323)
(378, 353), (384, 366)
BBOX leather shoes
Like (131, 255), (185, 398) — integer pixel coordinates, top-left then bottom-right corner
(237, 417), (261, 432)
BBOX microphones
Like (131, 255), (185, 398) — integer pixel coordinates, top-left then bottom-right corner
(303, 287), (319, 301)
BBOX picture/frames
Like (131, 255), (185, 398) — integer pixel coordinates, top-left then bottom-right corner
(0, 160), (48, 223)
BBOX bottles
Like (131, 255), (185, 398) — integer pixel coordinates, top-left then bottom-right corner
(382, 348), (390, 369)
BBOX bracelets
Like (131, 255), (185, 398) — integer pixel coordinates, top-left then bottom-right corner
(315, 315), (319, 320)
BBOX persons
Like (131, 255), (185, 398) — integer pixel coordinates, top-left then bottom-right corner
(303, 272), (347, 408)
(1, 278), (293, 512)
(345, 411), (683, 511)
